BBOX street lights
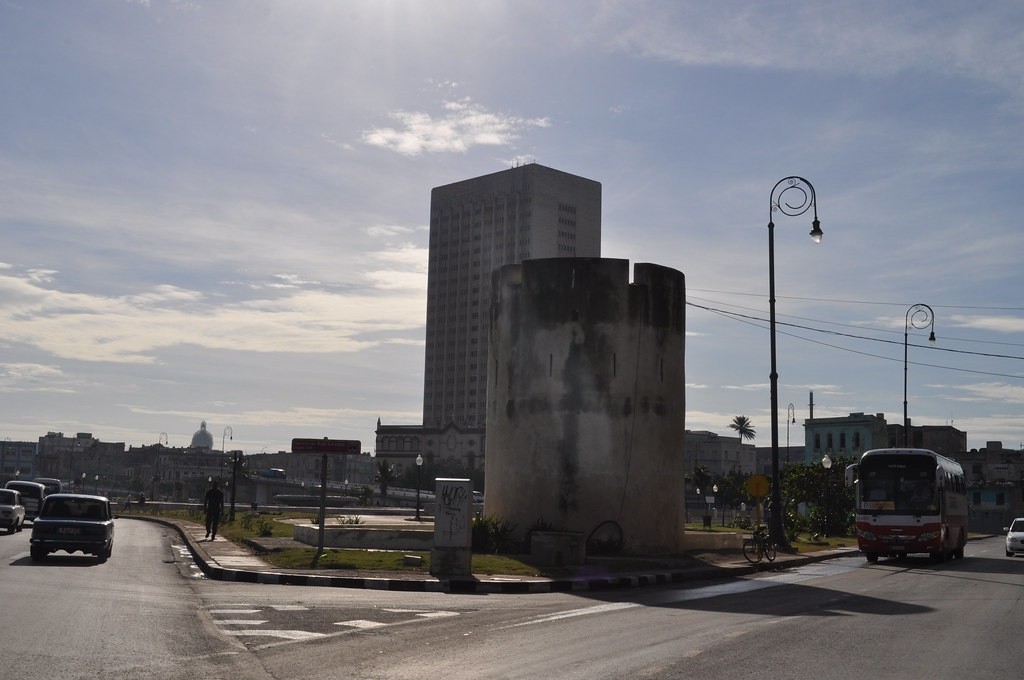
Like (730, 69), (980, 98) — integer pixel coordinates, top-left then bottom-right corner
(157, 432), (168, 473)
(82, 473), (86, 494)
(712, 484), (718, 508)
(301, 481), (304, 495)
(904, 304), (937, 448)
(787, 404), (796, 462)
(822, 455), (832, 538)
(208, 475), (212, 491)
(68, 434), (80, 485)
(767, 176), (823, 543)
(2, 437), (12, 479)
(226, 481), (229, 503)
(696, 488), (701, 507)
(221, 426), (232, 477)
(416, 455), (423, 517)
(94, 475), (98, 495)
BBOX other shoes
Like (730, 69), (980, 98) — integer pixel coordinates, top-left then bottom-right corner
(205, 531), (210, 537)
(212, 535), (214, 541)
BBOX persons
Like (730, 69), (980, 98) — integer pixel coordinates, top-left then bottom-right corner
(203, 481), (225, 540)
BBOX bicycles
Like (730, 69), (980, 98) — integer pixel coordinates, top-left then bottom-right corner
(742, 523), (776, 563)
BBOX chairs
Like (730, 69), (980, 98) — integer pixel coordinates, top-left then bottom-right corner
(869, 489), (888, 501)
(87, 504), (102, 518)
(2, 495), (11, 504)
(51, 503), (69, 516)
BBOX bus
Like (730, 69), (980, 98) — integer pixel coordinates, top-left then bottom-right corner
(845, 448), (968, 563)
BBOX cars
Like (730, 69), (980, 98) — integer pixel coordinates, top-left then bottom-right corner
(33, 478), (62, 496)
(5, 481), (45, 522)
(0, 489), (25, 534)
(30, 494), (119, 558)
(1003, 518), (1024, 556)
